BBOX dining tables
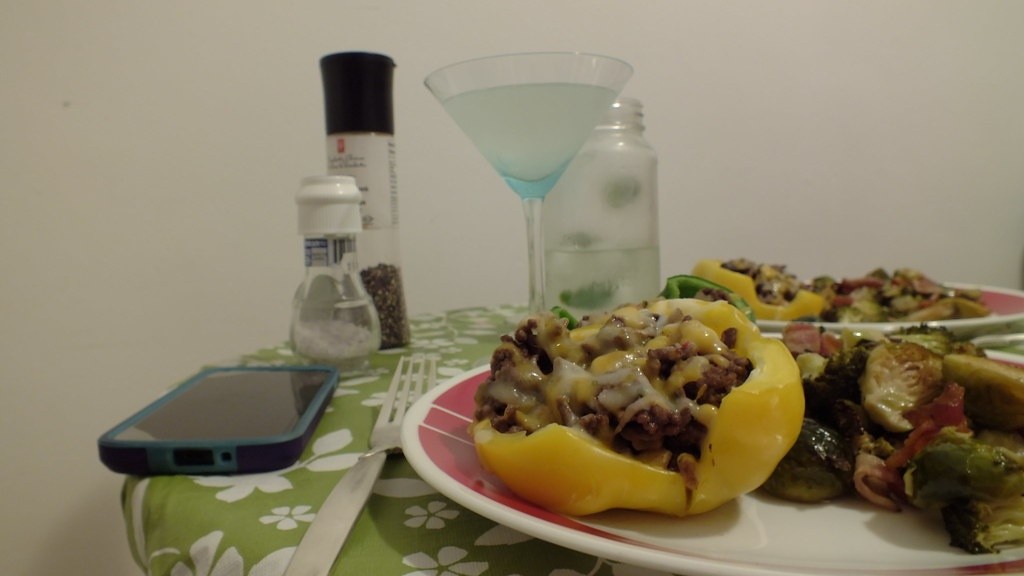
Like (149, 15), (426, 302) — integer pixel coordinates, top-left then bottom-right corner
(119, 303), (679, 576)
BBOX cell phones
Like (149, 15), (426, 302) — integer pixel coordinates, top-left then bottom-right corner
(97, 364), (339, 477)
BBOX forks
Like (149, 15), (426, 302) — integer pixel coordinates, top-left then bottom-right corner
(281, 354), (436, 576)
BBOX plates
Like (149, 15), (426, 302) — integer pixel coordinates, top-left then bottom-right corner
(400, 334), (1024, 575)
(754, 282), (1024, 332)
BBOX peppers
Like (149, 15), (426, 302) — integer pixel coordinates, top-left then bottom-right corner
(473, 258), (826, 516)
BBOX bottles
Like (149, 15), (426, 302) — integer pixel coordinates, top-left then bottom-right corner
(319, 49), (412, 352)
(286, 175), (382, 364)
(539, 96), (663, 311)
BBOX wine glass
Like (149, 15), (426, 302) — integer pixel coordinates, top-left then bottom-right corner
(424, 50), (634, 313)
